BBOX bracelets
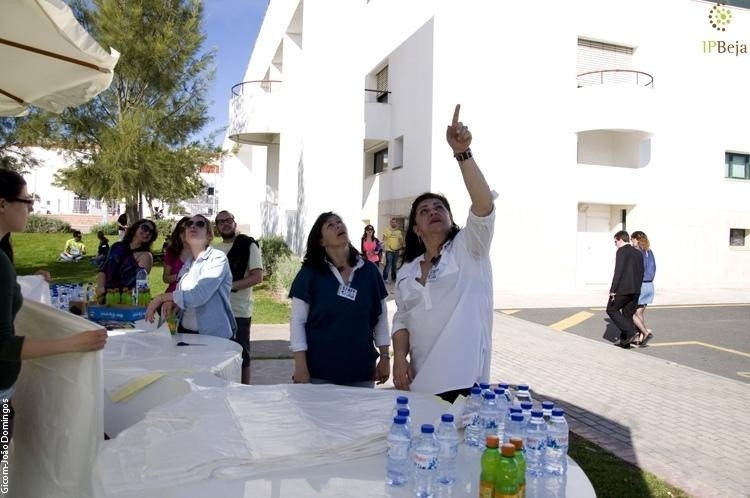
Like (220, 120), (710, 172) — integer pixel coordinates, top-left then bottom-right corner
(454, 148), (472, 161)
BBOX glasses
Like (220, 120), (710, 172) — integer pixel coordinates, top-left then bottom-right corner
(185, 221), (205, 228)
(216, 218), (233, 224)
(366, 228), (372, 231)
(16, 197), (35, 205)
(141, 224), (154, 234)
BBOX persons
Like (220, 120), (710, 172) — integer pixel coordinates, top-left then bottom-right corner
(95, 219), (157, 305)
(361, 224), (383, 267)
(212, 211), (263, 384)
(97, 231), (109, 255)
(382, 218), (404, 284)
(0, 169), (108, 464)
(162, 216), (192, 293)
(606, 230), (644, 348)
(391, 104), (496, 404)
(145, 215), (238, 340)
(630, 231), (656, 348)
(117, 213), (126, 240)
(287, 212), (390, 388)
(60, 231), (86, 263)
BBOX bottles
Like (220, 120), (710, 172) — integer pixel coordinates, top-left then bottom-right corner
(435, 414), (458, 487)
(50, 281), (98, 312)
(167, 305), (176, 335)
(136, 266), (149, 306)
(385, 395), (412, 486)
(410, 424), (439, 498)
(106, 288), (151, 307)
(457, 380), (569, 498)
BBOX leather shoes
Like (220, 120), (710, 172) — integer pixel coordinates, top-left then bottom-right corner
(614, 330), (653, 348)
